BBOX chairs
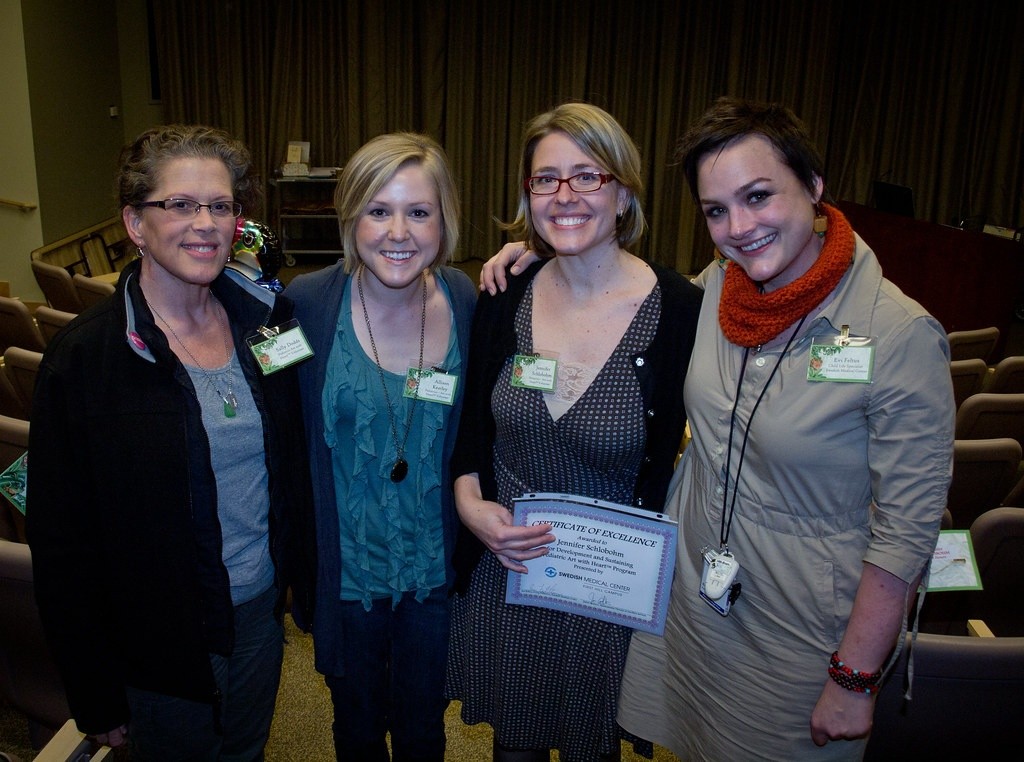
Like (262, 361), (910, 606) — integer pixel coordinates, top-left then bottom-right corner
(0, 260), (117, 726)
(865, 329), (1024, 762)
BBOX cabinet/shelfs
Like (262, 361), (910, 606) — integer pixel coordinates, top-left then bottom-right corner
(275, 174), (346, 267)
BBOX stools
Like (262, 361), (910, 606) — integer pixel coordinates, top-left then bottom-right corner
(90, 272), (122, 286)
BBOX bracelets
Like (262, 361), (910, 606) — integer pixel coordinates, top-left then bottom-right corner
(828, 652), (881, 694)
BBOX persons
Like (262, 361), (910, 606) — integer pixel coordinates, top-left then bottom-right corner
(28, 124), (302, 762)
(480, 99), (956, 762)
(449, 102), (703, 761)
(282, 132), (479, 762)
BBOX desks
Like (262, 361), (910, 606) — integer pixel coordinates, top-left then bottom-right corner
(837, 201), (1023, 329)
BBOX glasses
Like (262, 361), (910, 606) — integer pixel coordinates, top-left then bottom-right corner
(523, 172), (615, 195)
(141, 198), (242, 219)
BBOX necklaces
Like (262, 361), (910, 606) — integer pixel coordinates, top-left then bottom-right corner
(359, 261), (427, 482)
(138, 289), (238, 419)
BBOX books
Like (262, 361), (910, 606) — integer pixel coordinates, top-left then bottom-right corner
(288, 141), (337, 177)
